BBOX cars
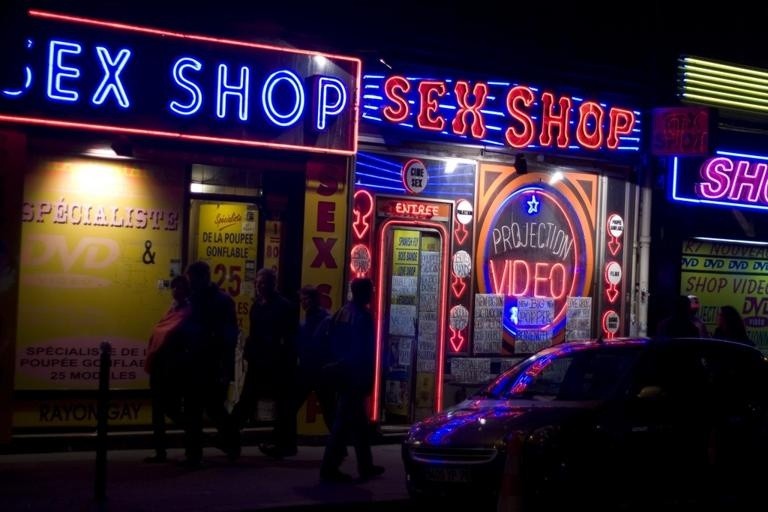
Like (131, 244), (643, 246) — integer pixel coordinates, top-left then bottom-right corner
(401, 333), (767, 510)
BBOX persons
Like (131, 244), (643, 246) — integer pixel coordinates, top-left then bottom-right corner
(653, 293), (760, 349)
(142, 260), (385, 487)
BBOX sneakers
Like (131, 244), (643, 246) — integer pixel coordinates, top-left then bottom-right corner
(359, 464), (384, 476)
(263, 443), (299, 458)
(319, 464), (353, 483)
